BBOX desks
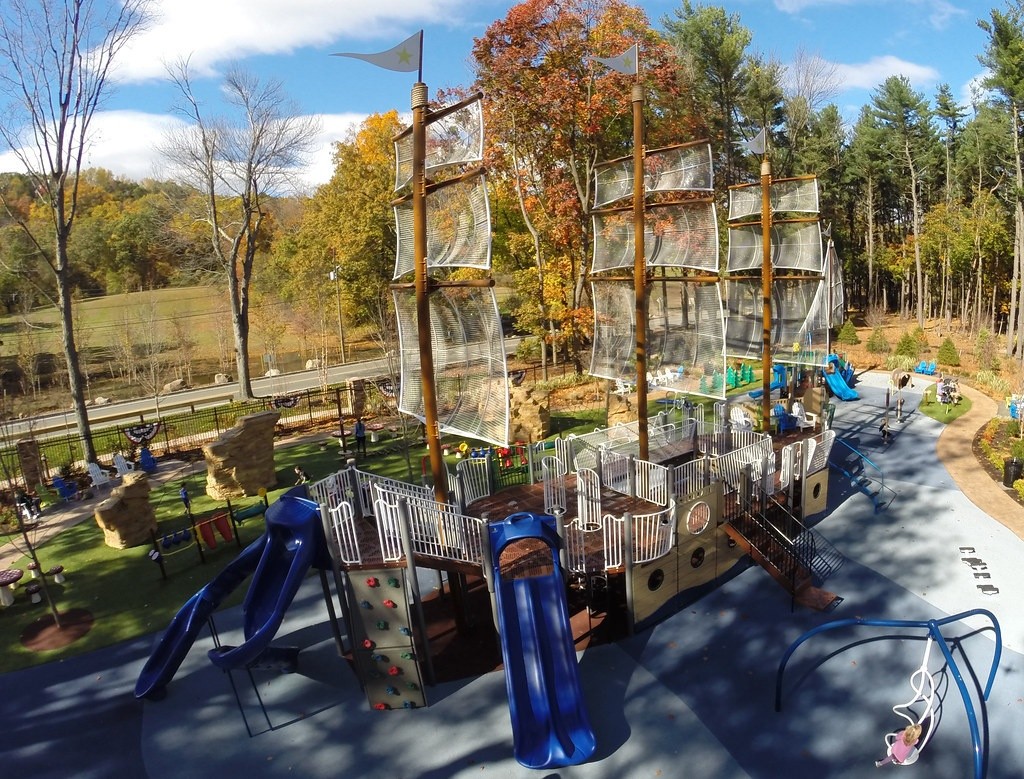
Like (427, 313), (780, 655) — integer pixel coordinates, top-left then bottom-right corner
(364, 423), (385, 443)
(331, 430), (352, 447)
(0, 568), (24, 606)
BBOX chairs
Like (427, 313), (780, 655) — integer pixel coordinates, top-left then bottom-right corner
(34, 483), (61, 508)
(1006, 393), (1024, 419)
(15, 496), (46, 520)
(727, 401), (818, 435)
(615, 366), (684, 396)
(111, 454), (135, 478)
(139, 448), (158, 473)
(52, 478), (79, 502)
(87, 462), (110, 489)
(914, 360), (927, 374)
(924, 361), (936, 376)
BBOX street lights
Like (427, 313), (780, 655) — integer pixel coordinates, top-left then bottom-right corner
(329, 249), (346, 363)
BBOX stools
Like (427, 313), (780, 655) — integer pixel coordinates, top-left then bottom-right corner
(25, 584), (42, 604)
(318, 441), (328, 451)
(27, 562), (41, 578)
(49, 565), (65, 584)
(388, 427), (399, 437)
(425, 440), (462, 458)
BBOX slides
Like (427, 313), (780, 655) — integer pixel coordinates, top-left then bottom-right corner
(133, 534), (268, 700)
(207, 535), (319, 673)
(748, 381), (782, 398)
(492, 571), (598, 769)
(823, 368), (858, 401)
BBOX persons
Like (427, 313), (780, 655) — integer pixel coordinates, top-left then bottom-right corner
(896, 397), (905, 422)
(936, 376), (960, 404)
(879, 419), (891, 440)
(354, 417), (367, 457)
(179, 482), (193, 519)
(16, 489), (45, 518)
(294, 465), (310, 485)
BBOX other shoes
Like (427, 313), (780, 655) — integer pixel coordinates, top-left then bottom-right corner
(184, 512), (187, 516)
(187, 514), (190, 518)
